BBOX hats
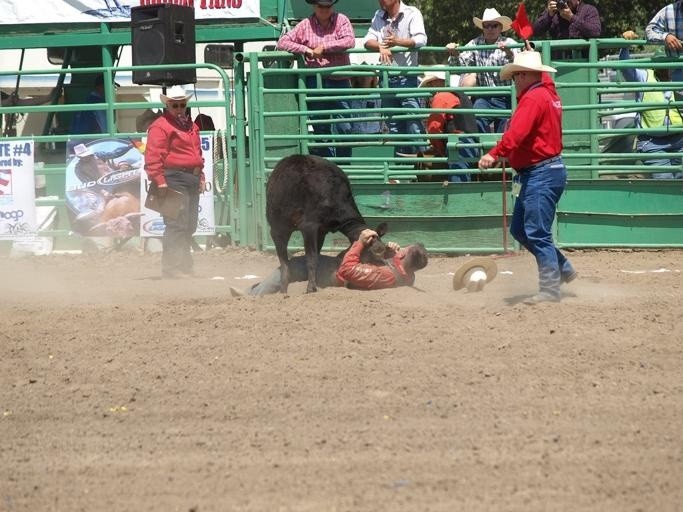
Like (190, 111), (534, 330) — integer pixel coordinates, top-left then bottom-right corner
(96, 76), (120, 87)
(160, 85), (192, 103)
(500, 51), (557, 81)
(473, 8), (512, 32)
(418, 65), (445, 87)
(305, 0), (337, 7)
(452, 258), (497, 292)
(69, 144), (94, 157)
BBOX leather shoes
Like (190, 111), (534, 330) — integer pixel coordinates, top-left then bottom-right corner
(524, 272), (576, 305)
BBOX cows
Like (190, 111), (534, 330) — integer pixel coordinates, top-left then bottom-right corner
(265, 154), (396, 294)
(396, 146), (511, 181)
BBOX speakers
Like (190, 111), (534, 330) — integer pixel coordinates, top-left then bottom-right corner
(131, 3), (197, 85)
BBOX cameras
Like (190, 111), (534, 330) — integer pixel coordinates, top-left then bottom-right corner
(556, 0), (566, 9)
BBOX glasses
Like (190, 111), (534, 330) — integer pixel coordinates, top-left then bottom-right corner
(483, 23), (498, 28)
(173, 104), (186, 108)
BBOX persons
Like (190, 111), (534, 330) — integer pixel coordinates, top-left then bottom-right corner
(276, 1), (355, 166)
(144, 85), (207, 280)
(341, 0), (683, 184)
(229, 230), (429, 300)
(68, 143), (134, 192)
(477, 51), (578, 305)
